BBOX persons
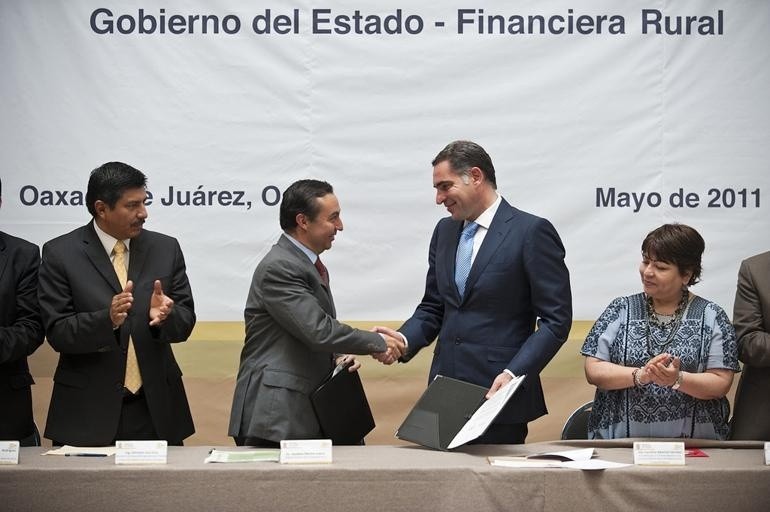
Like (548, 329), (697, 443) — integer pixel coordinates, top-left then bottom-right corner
(579, 223), (741, 441)
(36, 161), (198, 447)
(226, 179), (407, 448)
(0, 176), (45, 450)
(727, 248), (770, 441)
(371, 138), (572, 446)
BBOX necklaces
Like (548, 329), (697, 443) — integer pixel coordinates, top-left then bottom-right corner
(645, 283), (690, 329)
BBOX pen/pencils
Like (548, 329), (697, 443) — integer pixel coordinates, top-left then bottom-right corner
(209, 448), (215, 454)
(64, 454), (108, 456)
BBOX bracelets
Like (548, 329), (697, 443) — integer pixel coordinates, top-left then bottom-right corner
(670, 371), (683, 392)
(631, 365), (645, 391)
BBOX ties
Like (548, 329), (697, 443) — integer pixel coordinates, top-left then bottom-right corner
(111, 239), (142, 395)
(315, 256), (328, 288)
(455, 222), (479, 301)
